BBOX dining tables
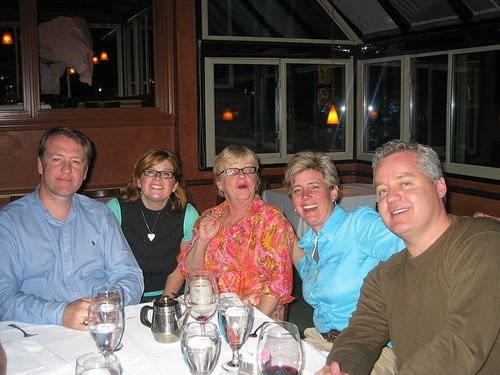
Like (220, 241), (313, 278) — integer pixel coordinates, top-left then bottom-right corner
(0, 297), (334, 375)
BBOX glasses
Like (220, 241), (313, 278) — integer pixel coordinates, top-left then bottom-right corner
(217, 166), (257, 176)
(143, 169), (175, 179)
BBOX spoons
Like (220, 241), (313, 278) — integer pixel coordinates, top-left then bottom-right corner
(249, 322), (266, 338)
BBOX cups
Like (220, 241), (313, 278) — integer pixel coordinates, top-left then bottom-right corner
(256, 321), (303, 375)
(189, 280), (213, 304)
(180, 321), (221, 375)
(75, 352), (120, 375)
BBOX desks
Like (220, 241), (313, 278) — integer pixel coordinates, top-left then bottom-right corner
(261, 182), (378, 242)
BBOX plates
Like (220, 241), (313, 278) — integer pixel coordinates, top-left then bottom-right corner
(180, 298), (219, 310)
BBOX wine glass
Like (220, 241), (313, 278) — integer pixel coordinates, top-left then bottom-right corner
(184, 271), (255, 372)
(87, 301), (125, 368)
(91, 283), (125, 351)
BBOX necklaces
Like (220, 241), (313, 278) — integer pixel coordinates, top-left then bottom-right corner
(141, 198), (169, 241)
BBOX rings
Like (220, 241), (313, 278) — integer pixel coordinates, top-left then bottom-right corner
(83, 320), (88, 325)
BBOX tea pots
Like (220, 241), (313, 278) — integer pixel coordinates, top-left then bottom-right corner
(140, 295), (191, 343)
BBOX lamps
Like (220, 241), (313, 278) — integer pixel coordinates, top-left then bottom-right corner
(327, 0), (340, 124)
(1, 32), (109, 73)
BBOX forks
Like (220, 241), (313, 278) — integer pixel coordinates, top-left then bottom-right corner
(7, 324), (39, 337)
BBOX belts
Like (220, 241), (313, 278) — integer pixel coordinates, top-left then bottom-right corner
(320, 330), (340, 342)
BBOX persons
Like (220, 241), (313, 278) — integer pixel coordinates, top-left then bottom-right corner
(0, 127), (144, 331)
(107, 147), (200, 306)
(282, 141), (500, 375)
(177, 145), (294, 324)
